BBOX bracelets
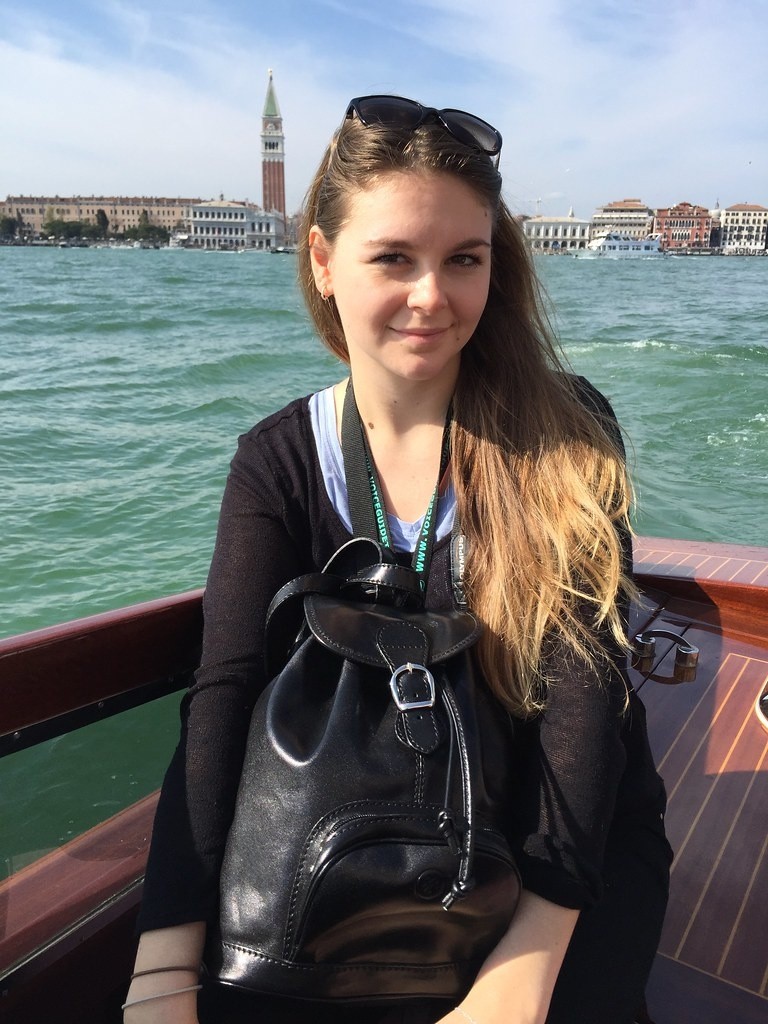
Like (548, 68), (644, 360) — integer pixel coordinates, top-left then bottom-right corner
(131, 965), (202, 981)
(455, 1007), (476, 1024)
(121, 984), (203, 1009)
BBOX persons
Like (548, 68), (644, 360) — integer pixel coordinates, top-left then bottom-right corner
(124, 94), (675, 1024)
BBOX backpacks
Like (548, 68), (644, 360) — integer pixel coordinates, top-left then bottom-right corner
(205, 535), (524, 1008)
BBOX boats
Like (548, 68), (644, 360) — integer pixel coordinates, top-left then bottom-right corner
(564, 229), (666, 260)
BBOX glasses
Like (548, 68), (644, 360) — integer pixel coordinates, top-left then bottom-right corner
(337, 95), (504, 173)
